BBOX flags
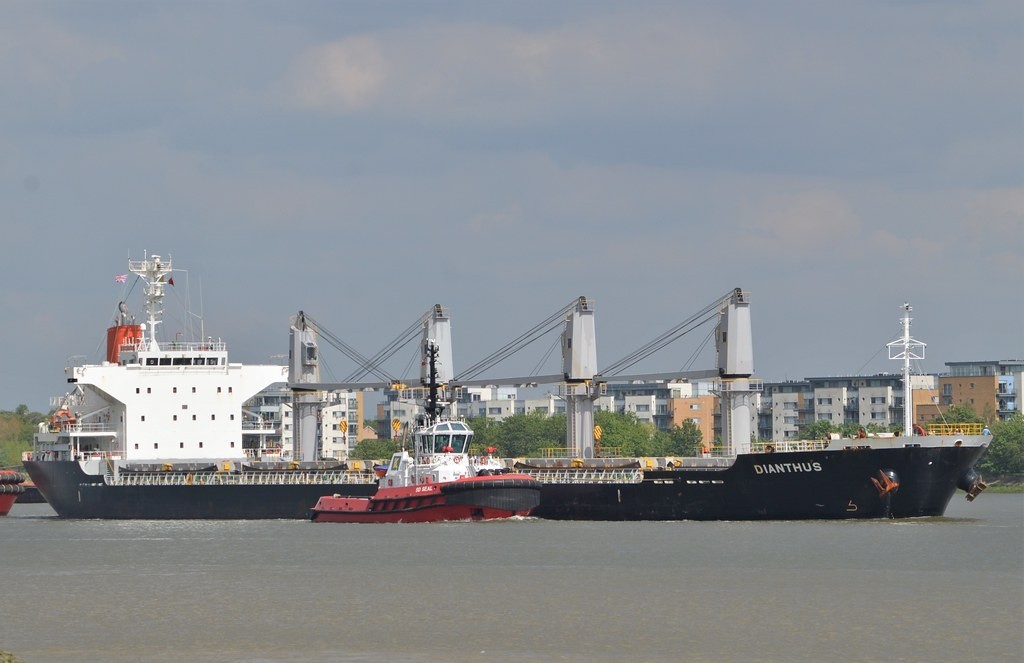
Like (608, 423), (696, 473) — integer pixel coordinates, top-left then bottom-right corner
(115, 274), (128, 284)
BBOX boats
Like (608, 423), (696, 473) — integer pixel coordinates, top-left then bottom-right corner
(307, 414), (545, 526)
(21, 244), (506, 523)
(477, 296), (994, 524)
(0, 469), (26, 517)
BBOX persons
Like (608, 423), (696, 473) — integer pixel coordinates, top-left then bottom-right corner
(765, 446), (774, 453)
